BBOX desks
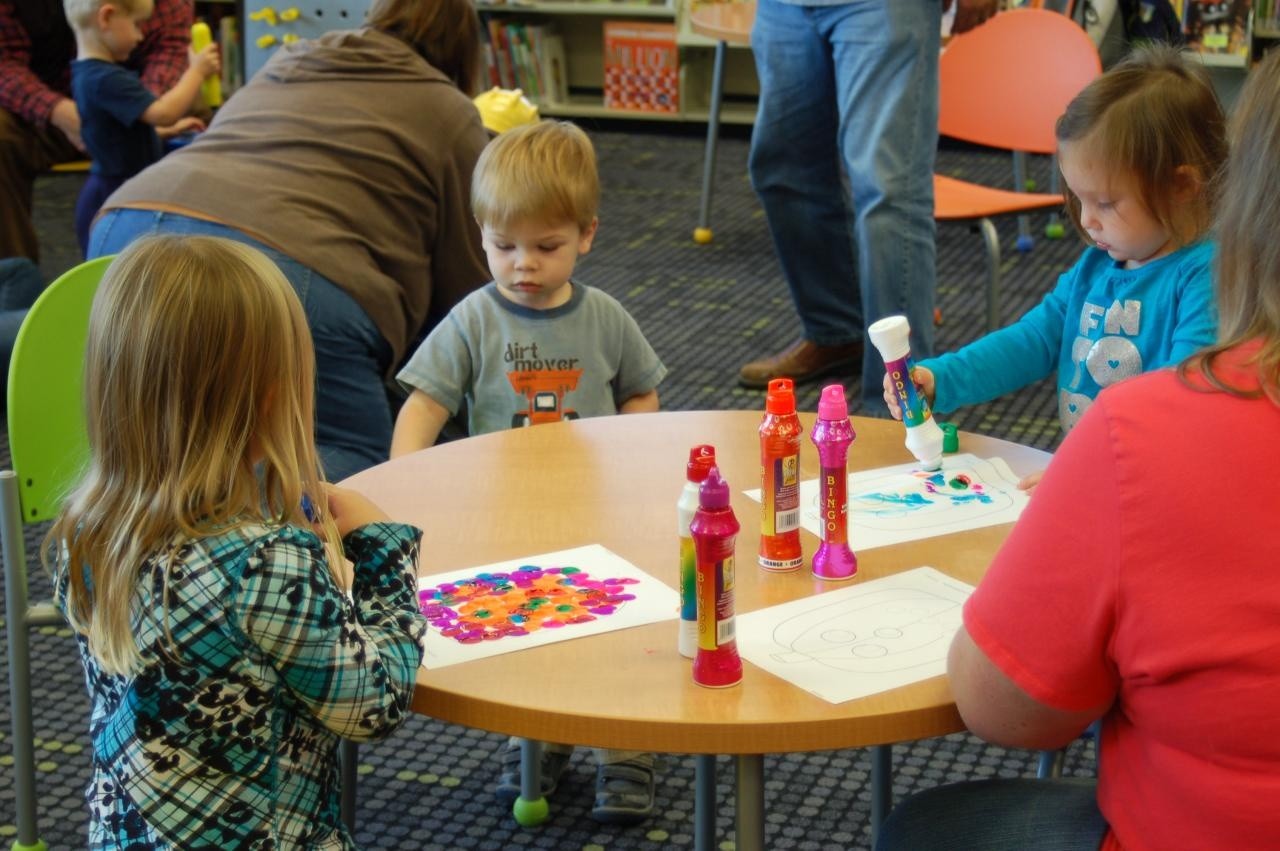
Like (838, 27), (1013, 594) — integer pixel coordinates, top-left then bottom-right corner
(685, 0), (768, 246)
(314, 408), (1103, 851)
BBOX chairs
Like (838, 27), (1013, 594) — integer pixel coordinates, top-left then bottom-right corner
(890, 4), (1112, 391)
(1, 249), (294, 851)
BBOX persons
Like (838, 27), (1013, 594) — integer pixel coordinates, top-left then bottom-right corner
(82, 0), (504, 488)
(389, 119), (671, 826)
(887, 39), (1230, 459)
(63, 0), (222, 264)
(871, 36), (1279, 851)
(729, 1), (947, 431)
(1, 0), (209, 281)
(35, 232), (432, 851)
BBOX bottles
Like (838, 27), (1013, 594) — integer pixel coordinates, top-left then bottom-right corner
(809, 384), (857, 580)
(678, 442), (716, 659)
(867, 315), (943, 471)
(689, 465), (744, 688)
(190, 16), (221, 106)
(758, 378), (805, 572)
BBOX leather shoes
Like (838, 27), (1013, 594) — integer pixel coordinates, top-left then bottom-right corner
(738, 336), (865, 391)
